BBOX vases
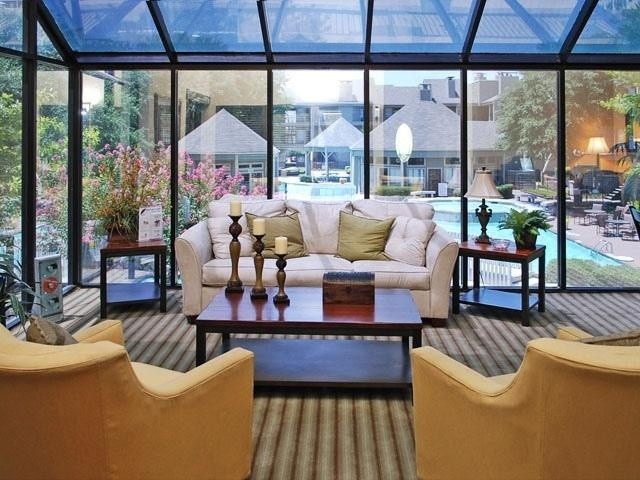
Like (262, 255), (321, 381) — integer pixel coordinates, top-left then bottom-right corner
(104, 220), (138, 240)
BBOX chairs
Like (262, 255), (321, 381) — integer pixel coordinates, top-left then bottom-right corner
(613, 206), (628, 220)
(0, 322), (254, 479)
(406, 325), (638, 478)
(593, 204), (602, 210)
(597, 214), (606, 227)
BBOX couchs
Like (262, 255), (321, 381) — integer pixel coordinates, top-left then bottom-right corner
(174, 194), (458, 330)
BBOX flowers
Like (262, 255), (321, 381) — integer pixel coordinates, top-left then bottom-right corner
(93, 161), (158, 228)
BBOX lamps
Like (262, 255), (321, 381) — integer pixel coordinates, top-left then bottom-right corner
(462, 169), (505, 242)
(586, 135), (611, 165)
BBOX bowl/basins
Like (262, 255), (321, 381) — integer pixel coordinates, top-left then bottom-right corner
(489, 239), (510, 251)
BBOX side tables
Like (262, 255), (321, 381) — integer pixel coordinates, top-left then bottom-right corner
(451, 239), (547, 329)
(101, 239), (168, 322)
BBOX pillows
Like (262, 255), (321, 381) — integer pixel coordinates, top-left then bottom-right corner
(24, 311), (76, 345)
(207, 212), (308, 262)
(335, 211), (436, 267)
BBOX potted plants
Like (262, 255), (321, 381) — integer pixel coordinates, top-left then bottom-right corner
(497, 207), (554, 250)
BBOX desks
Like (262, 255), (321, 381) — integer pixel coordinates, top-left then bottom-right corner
(584, 209), (605, 225)
(604, 219), (630, 236)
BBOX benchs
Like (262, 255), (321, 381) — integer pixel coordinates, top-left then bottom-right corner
(410, 190), (436, 198)
